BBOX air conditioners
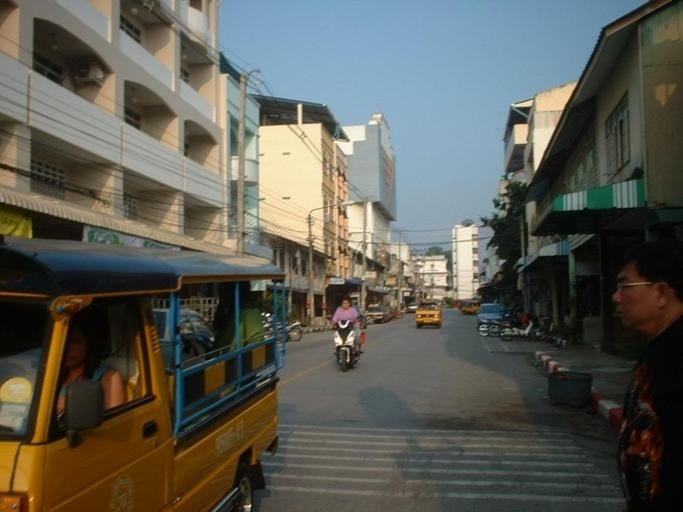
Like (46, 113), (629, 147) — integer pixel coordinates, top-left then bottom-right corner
(72, 57), (103, 82)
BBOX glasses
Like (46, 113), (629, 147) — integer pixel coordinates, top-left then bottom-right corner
(616, 280), (653, 294)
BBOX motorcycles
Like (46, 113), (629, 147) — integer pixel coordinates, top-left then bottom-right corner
(476, 320), (544, 341)
(332, 320), (363, 371)
(262, 311), (303, 342)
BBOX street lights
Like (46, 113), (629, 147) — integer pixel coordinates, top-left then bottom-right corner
(307, 200), (354, 323)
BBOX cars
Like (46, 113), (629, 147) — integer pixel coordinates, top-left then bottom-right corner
(408, 299), (443, 329)
(463, 301), (502, 323)
(365, 303), (392, 323)
(152, 306), (208, 333)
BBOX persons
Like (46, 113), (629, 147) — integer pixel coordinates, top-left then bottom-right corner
(330, 295), (363, 355)
(43, 311), (125, 429)
(608, 237), (682, 512)
(213, 280), (268, 381)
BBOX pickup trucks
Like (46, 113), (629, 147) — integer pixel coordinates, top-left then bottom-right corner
(0, 233), (286, 512)
